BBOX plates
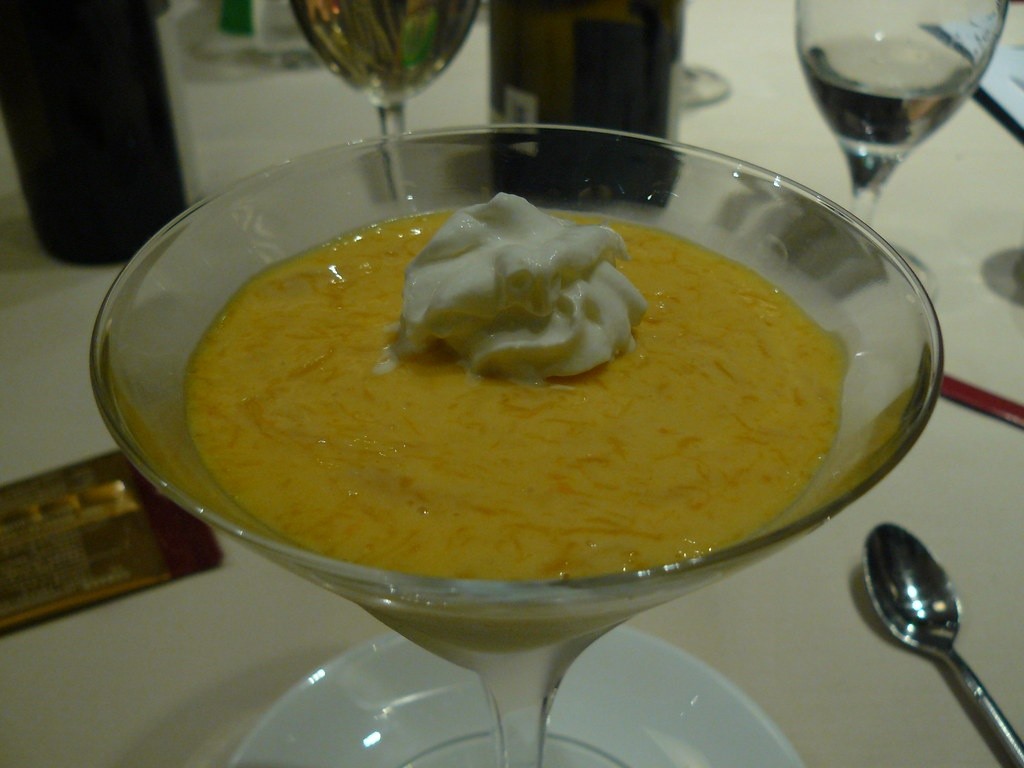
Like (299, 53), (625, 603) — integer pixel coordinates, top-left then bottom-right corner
(228, 622), (806, 768)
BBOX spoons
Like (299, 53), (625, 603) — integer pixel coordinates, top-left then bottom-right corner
(863, 520), (1024, 768)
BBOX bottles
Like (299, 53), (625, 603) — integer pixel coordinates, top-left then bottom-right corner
(0, 0), (186, 268)
(489, 0), (686, 212)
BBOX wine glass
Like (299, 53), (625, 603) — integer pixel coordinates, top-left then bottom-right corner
(91, 122), (944, 768)
(286, 0), (482, 204)
(792, 0), (1009, 306)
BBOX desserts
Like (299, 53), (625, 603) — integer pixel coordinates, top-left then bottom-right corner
(392, 190), (646, 381)
